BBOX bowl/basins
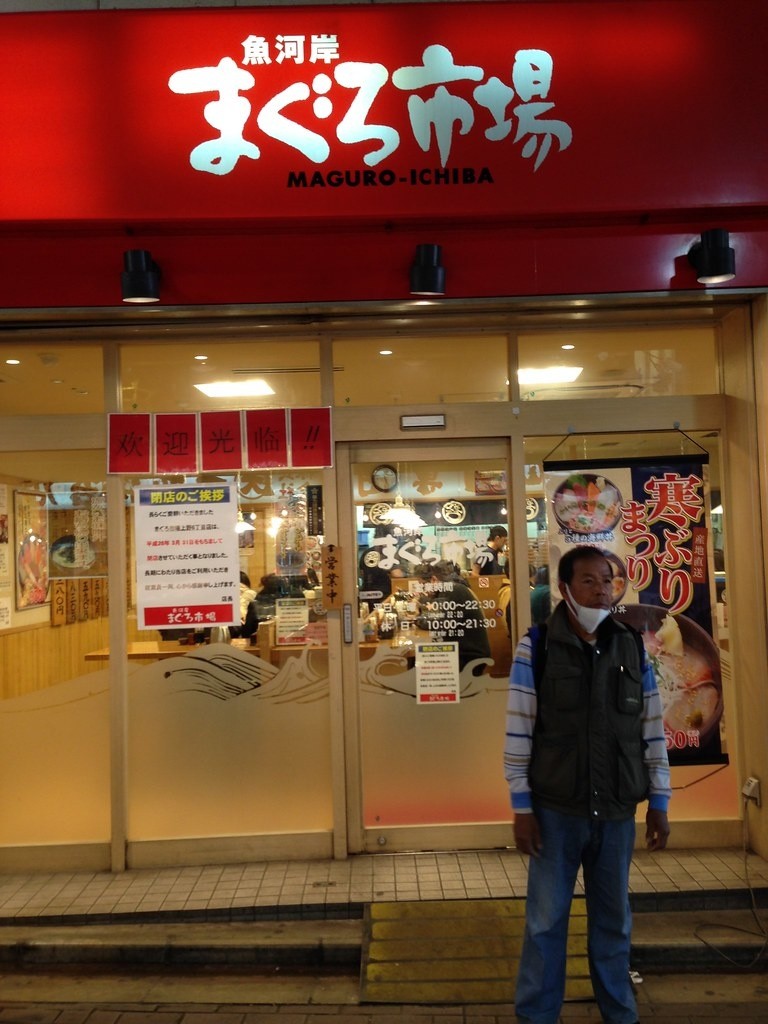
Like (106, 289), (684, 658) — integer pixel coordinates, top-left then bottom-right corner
(552, 473), (624, 534)
(611, 603), (723, 749)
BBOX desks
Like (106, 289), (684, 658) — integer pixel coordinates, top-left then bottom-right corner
(84, 590), (432, 706)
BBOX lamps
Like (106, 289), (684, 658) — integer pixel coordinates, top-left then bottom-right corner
(409, 244), (446, 296)
(690, 228), (736, 284)
(235, 472), (256, 534)
(379, 462), (429, 529)
(119, 249), (161, 303)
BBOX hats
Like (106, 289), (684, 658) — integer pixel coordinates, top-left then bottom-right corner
(487, 526), (507, 542)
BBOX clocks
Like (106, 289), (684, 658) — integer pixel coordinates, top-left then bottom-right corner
(371, 464), (398, 493)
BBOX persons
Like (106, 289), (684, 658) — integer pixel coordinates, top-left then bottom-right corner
(413, 560), (491, 676)
(359, 531), (403, 613)
(503, 545), (670, 1024)
(497, 557), (549, 638)
(158, 568), (320, 649)
(472, 525), (507, 576)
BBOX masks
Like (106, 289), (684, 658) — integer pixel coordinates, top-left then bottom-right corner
(565, 584), (612, 634)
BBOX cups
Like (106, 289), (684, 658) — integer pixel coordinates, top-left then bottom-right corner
(359, 593), (415, 642)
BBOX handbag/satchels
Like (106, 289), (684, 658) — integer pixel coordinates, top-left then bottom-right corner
(210, 625), (231, 644)
